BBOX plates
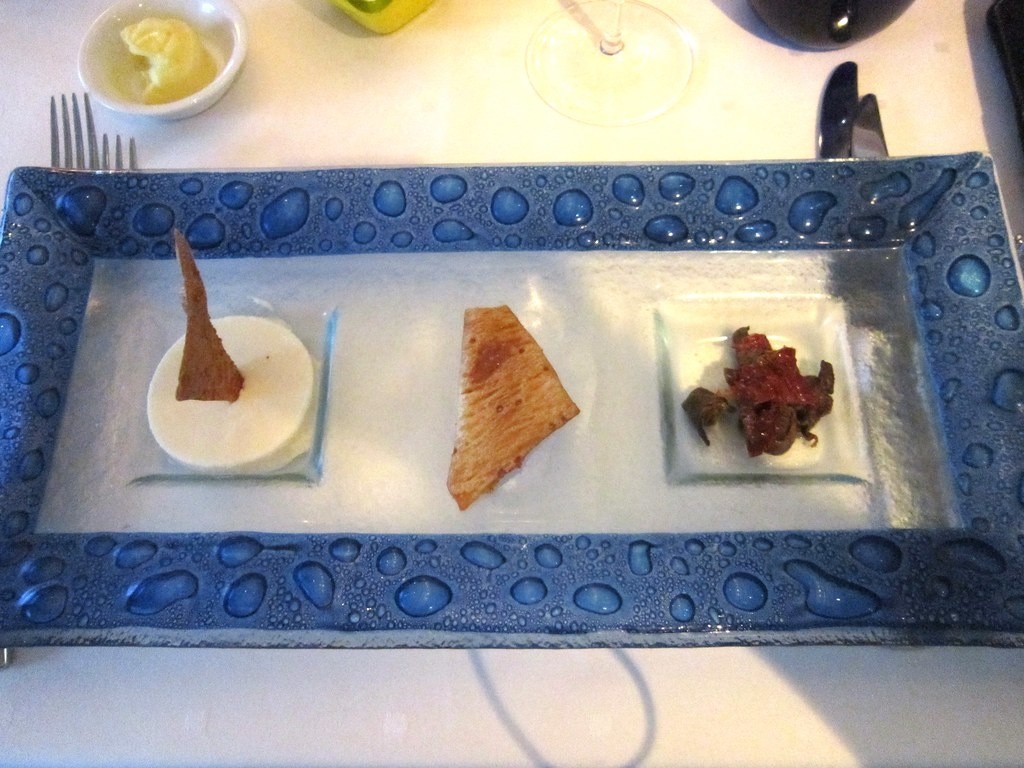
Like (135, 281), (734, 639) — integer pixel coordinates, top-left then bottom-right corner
(0, 153), (1024, 648)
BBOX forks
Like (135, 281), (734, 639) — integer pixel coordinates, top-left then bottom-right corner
(51, 93), (99, 170)
(90, 132), (137, 170)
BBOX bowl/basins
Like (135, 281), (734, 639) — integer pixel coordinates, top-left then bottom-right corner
(78, 0), (248, 121)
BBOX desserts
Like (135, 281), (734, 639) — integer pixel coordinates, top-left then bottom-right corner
(146, 228), (316, 473)
(446, 304), (582, 511)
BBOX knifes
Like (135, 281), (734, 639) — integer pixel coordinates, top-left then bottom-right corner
(816, 61), (859, 158)
(851, 93), (889, 158)
